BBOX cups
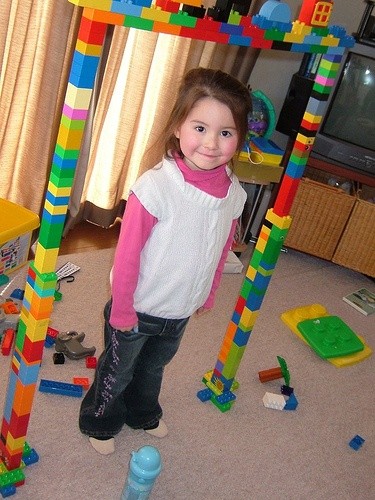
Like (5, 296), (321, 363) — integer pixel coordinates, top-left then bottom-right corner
(120, 445), (162, 500)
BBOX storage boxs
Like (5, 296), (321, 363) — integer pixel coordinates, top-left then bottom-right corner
(280, 156), (375, 278)
(0, 197), (40, 274)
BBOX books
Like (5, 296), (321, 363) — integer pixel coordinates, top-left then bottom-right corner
(343, 287), (375, 316)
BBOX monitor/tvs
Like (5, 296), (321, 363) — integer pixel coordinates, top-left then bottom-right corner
(309, 42), (375, 178)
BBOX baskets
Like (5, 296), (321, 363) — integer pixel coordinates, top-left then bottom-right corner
(332, 195), (375, 278)
(281, 172), (355, 263)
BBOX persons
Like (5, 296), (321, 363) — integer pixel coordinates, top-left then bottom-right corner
(79, 68), (254, 455)
